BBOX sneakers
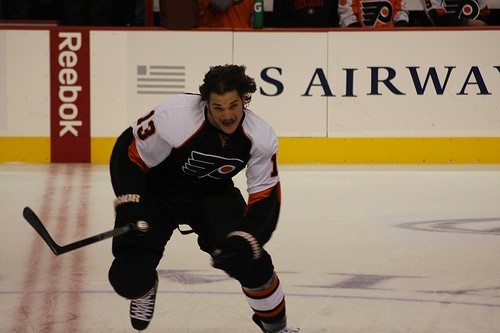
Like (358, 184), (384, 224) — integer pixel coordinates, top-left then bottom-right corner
(129, 270), (159, 330)
(253, 313), (301, 333)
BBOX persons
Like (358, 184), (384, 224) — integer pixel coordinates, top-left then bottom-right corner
(107, 65), (300, 333)
(152, 0), (500, 29)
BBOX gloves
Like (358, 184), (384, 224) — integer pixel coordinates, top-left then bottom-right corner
(112, 195), (147, 258)
(211, 231), (260, 271)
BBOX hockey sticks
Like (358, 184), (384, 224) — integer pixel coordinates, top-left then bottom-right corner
(22, 206), (131, 256)
